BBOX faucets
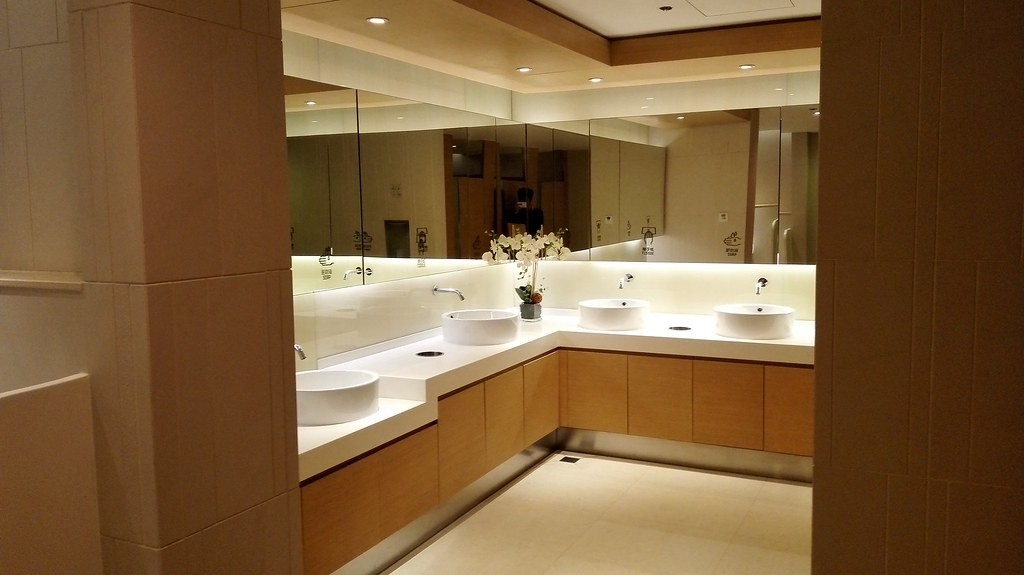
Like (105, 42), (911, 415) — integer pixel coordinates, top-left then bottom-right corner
(341, 269), (358, 281)
(294, 344), (307, 361)
(433, 284), (464, 301)
(756, 279), (769, 294)
(619, 274), (634, 289)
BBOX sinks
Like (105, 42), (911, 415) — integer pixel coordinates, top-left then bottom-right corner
(712, 303), (795, 338)
(441, 309), (521, 346)
(296, 368), (379, 426)
(578, 299), (649, 329)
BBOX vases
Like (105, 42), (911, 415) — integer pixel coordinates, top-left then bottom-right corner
(521, 302), (542, 319)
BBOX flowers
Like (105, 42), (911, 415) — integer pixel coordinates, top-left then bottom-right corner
(500, 228), (572, 293)
(481, 229), (514, 265)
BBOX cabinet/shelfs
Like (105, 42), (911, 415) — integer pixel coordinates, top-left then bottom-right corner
(590, 135), (666, 249)
(299, 347), (814, 574)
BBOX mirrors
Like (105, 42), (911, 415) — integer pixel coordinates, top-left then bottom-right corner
(284, 75), (820, 298)
